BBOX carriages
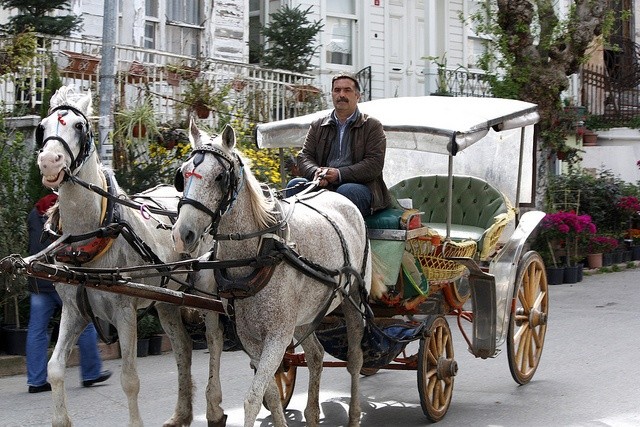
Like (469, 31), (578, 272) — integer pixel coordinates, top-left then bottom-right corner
(0, 88), (551, 426)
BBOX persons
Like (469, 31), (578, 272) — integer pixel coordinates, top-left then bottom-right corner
(287, 73), (389, 217)
(23, 193), (113, 392)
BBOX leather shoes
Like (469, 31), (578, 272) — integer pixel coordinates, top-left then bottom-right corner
(82, 370), (113, 387)
(27, 383), (51, 393)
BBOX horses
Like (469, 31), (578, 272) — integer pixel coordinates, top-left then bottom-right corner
(35, 84), (228, 427)
(169, 111), (373, 427)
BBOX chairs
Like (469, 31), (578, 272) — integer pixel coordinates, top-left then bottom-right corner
(385, 172), (515, 301)
